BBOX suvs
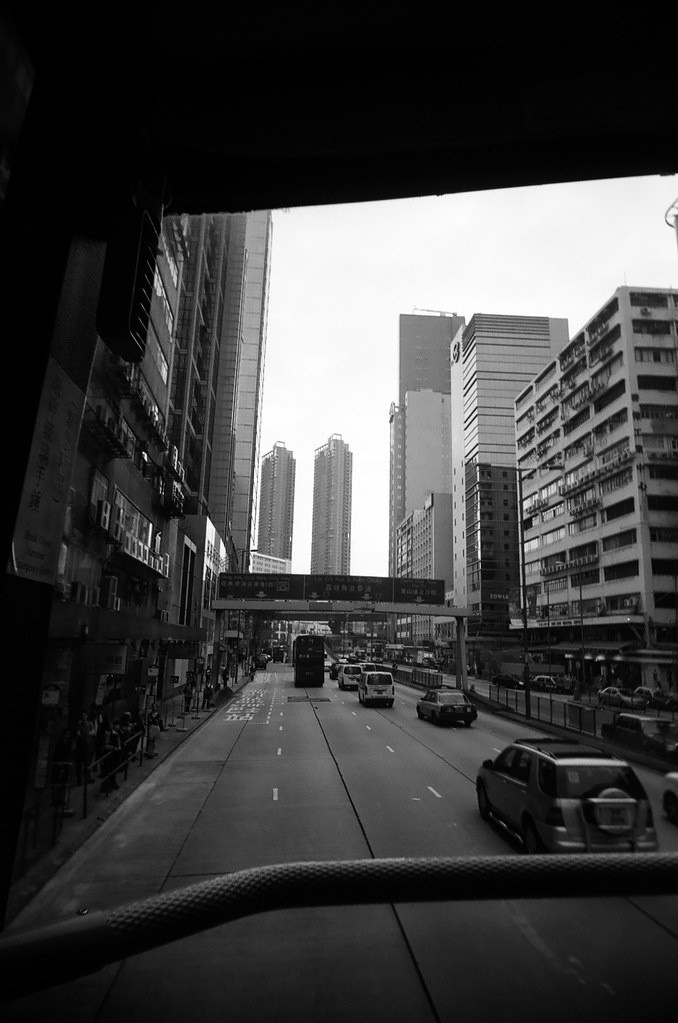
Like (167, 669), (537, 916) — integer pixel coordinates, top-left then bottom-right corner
(475, 737), (660, 855)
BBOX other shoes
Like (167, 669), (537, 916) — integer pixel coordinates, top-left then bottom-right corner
(83, 777), (95, 784)
(93, 764), (98, 773)
(111, 781), (120, 789)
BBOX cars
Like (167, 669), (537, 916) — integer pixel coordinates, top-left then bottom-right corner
(354, 663), (376, 672)
(601, 713), (678, 765)
(492, 673), (525, 690)
(598, 687), (678, 710)
(328, 663), (348, 680)
(529, 676), (575, 695)
(350, 648), (366, 660)
(660, 771), (678, 827)
(256, 654), (272, 670)
(416, 687), (478, 726)
(324, 661), (332, 672)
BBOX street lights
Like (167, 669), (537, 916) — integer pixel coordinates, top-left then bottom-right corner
(235, 550), (258, 684)
(556, 559), (585, 685)
(475, 462), (565, 719)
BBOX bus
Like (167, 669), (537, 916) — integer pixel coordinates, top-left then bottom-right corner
(367, 642), (384, 662)
(294, 635), (327, 688)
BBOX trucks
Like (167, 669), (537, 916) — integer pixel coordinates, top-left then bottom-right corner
(273, 647), (284, 663)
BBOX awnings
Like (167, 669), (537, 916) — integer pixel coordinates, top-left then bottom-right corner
(584, 640), (634, 651)
(550, 640), (584, 651)
(528, 644), (549, 651)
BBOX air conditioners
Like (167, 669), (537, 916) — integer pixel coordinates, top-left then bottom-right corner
(93, 404), (131, 454)
(71, 576), (119, 609)
(525, 499), (547, 513)
(99, 499), (110, 529)
(570, 498), (599, 516)
(558, 451), (632, 498)
(624, 597), (636, 608)
(540, 555), (595, 576)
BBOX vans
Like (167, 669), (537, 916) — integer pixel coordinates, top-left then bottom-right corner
(356, 672), (395, 708)
(337, 665), (363, 690)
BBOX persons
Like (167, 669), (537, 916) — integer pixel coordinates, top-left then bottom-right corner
(201, 683), (213, 710)
(249, 666), (256, 679)
(74, 706), (138, 797)
(438, 656), (449, 673)
(390, 655), (392, 660)
(392, 662), (398, 675)
(183, 682), (193, 713)
(220, 663), (229, 688)
(144, 702), (164, 737)
